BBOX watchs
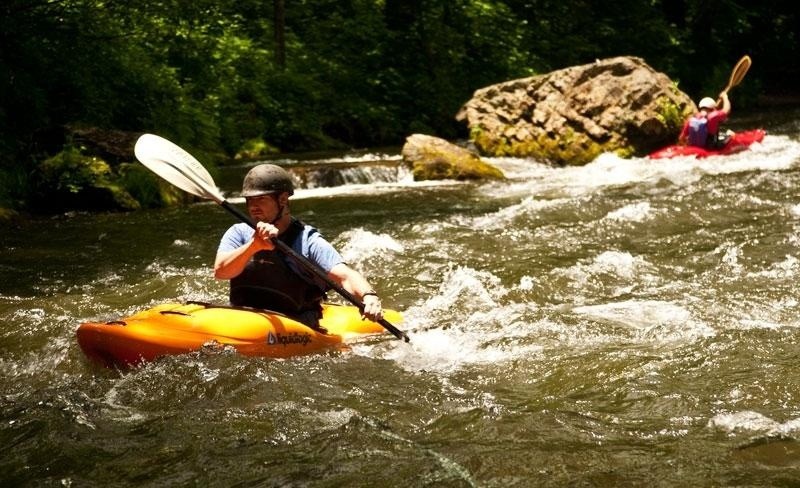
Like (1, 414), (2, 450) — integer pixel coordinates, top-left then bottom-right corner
(360, 288), (377, 301)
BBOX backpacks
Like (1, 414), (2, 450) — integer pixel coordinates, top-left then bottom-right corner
(687, 111), (708, 148)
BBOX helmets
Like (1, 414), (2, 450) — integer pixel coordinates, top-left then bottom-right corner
(240, 164), (294, 197)
(698, 97), (717, 110)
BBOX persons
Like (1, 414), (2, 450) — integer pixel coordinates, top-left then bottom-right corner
(214, 164), (384, 333)
(679, 91), (731, 150)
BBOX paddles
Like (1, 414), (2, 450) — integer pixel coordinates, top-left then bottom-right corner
(134, 134), (410, 341)
(716, 55), (752, 108)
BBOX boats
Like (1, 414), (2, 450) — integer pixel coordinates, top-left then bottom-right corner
(644, 129), (767, 161)
(74, 298), (405, 370)
